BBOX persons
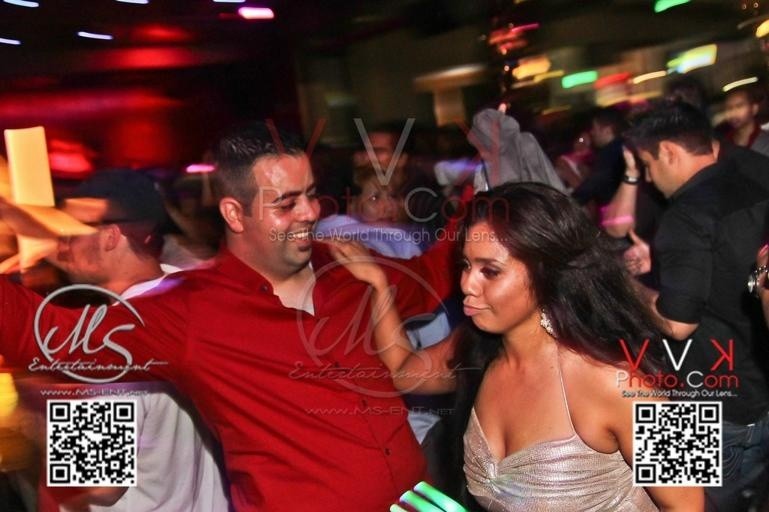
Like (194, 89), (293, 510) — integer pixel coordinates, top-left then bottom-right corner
(0, 87), (769, 512)
